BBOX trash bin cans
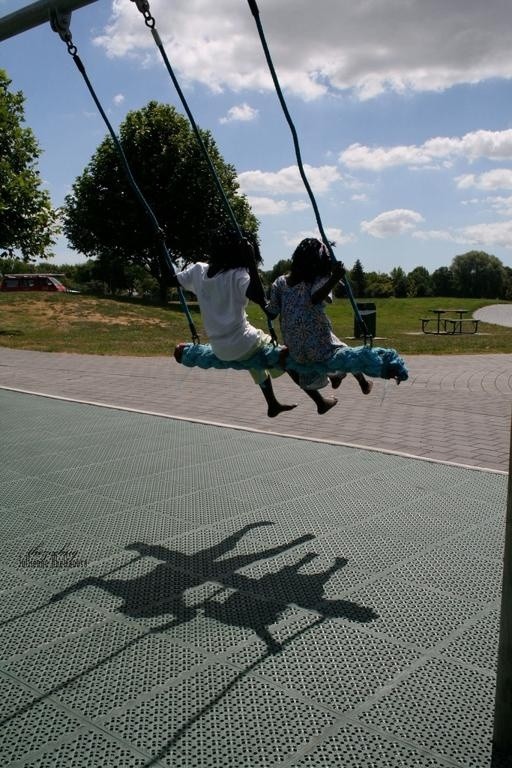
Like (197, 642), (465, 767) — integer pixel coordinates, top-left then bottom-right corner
(354, 303), (377, 338)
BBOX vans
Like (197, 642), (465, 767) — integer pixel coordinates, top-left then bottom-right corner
(2, 274), (66, 293)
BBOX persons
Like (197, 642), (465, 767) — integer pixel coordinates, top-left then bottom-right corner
(153, 226), (338, 417)
(261, 238), (373, 395)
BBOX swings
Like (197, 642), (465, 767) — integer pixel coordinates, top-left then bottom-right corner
(141, 0), (408, 375)
(51, 0), (294, 369)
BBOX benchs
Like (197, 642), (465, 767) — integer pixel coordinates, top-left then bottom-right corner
(444, 319), (482, 335)
(419, 317), (457, 334)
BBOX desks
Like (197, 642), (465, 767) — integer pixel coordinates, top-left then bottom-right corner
(428, 308), (469, 336)
